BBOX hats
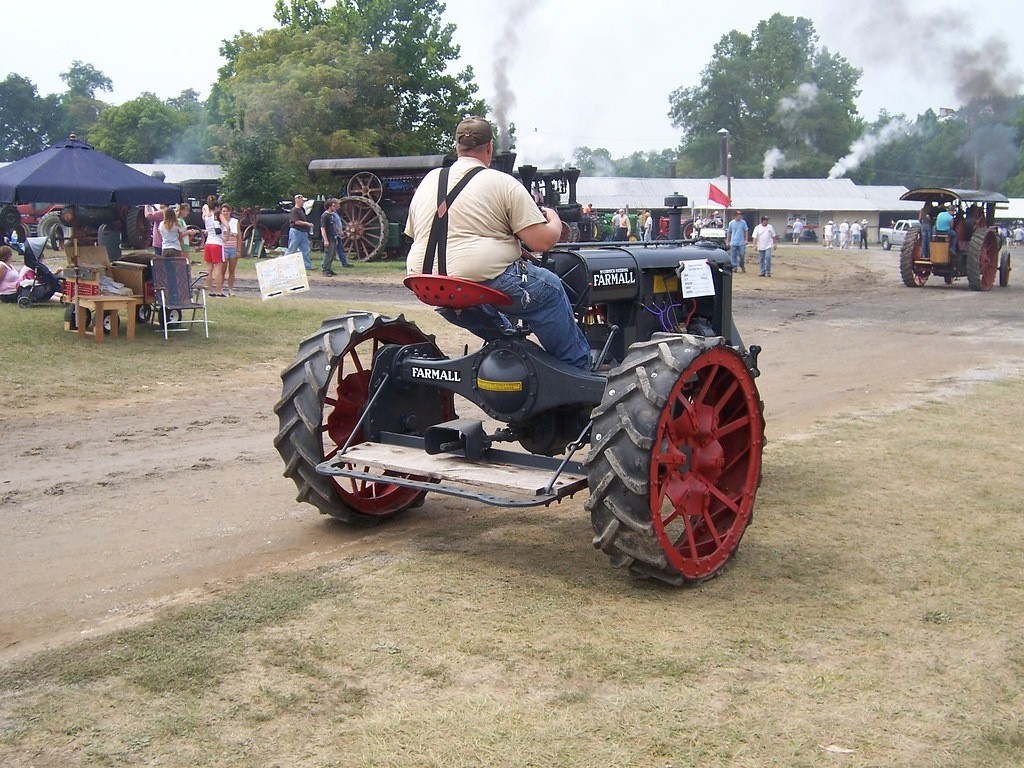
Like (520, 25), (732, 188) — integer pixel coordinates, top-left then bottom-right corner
(827, 221), (833, 224)
(861, 220), (868, 224)
(761, 216), (770, 220)
(295, 195), (307, 200)
(455, 115), (495, 146)
(947, 205), (956, 211)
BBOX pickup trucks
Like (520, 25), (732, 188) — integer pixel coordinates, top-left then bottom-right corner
(879, 219), (922, 250)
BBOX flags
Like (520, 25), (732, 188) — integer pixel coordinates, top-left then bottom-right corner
(708, 183), (732, 208)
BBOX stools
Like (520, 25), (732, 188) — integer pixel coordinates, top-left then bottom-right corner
(403, 274), (532, 339)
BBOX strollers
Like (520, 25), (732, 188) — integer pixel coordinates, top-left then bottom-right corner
(4, 236), (66, 309)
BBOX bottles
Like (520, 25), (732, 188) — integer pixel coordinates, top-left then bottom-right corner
(10, 230), (18, 244)
(309, 225), (314, 235)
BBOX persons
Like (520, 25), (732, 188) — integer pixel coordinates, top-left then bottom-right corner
(785, 218), (869, 250)
(0, 193), (354, 303)
(752, 216), (777, 277)
(918, 197), (958, 261)
(998, 224), (1024, 250)
(402, 116), (611, 372)
(581, 204), (724, 245)
(726, 211), (749, 274)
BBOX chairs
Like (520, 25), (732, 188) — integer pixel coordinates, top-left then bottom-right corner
(62, 244), (148, 340)
(149, 256), (208, 339)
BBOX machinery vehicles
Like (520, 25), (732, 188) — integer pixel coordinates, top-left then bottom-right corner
(601, 204), (641, 241)
(899, 188), (1012, 292)
(15, 201), (73, 250)
(655, 216), (699, 241)
(307, 151), (583, 263)
(568, 214), (607, 243)
(273, 192), (768, 588)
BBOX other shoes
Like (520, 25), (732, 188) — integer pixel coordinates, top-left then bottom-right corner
(208, 293), (216, 297)
(305, 270), (311, 275)
(950, 248), (956, 256)
(742, 267), (746, 273)
(759, 274), (765, 277)
(228, 293), (236, 297)
(732, 270), (737, 273)
(216, 293), (227, 298)
(766, 274), (771, 277)
(343, 264), (354, 268)
(324, 271), (337, 276)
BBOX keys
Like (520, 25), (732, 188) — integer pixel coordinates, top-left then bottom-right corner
(520, 269), (528, 283)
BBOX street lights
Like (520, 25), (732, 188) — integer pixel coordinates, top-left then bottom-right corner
(717, 128), (729, 176)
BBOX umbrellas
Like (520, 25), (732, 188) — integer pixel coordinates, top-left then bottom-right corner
(0, 132), (184, 328)
